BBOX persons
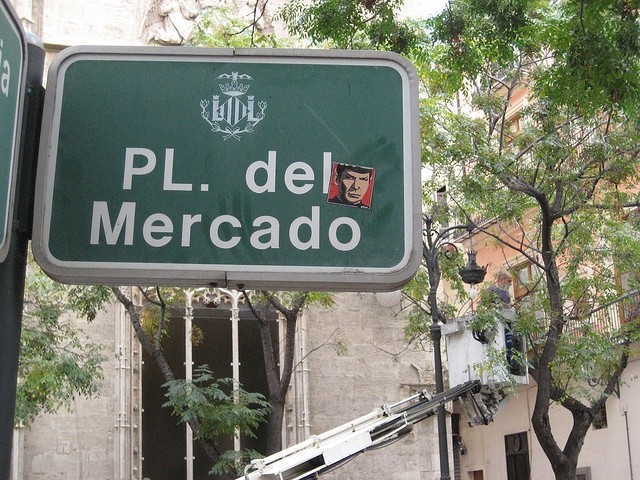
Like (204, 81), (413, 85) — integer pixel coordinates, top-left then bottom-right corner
(484, 272), (513, 373)
(328, 164), (372, 208)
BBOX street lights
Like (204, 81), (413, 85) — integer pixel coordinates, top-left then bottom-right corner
(413, 220), (488, 479)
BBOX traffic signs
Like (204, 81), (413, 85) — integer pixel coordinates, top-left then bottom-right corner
(34, 44), (419, 287)
(1, 3), (30, 264)
